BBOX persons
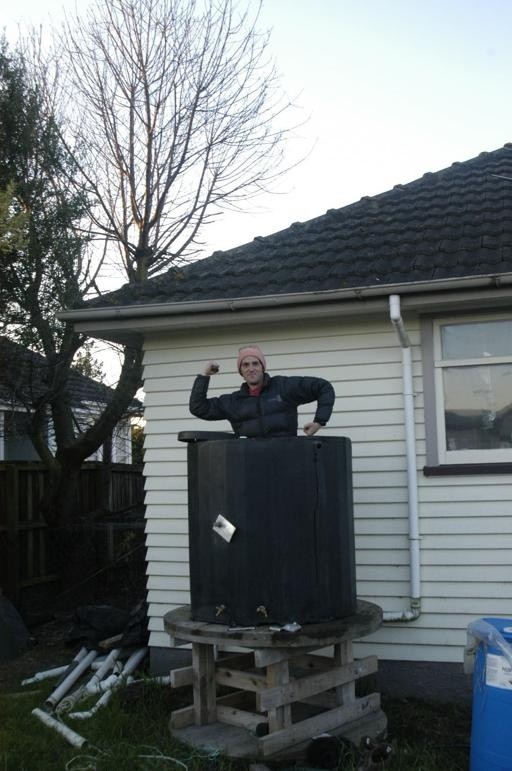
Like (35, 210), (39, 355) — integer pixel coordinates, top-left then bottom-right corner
(188, 343), (337, 438)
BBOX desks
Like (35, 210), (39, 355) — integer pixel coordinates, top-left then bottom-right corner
(163, 598), (382, 757)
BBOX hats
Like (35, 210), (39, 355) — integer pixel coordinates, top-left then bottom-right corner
(238, 345), (266, 377)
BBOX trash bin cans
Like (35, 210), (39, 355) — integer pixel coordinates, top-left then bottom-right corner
(470, 618), (511, 770)
